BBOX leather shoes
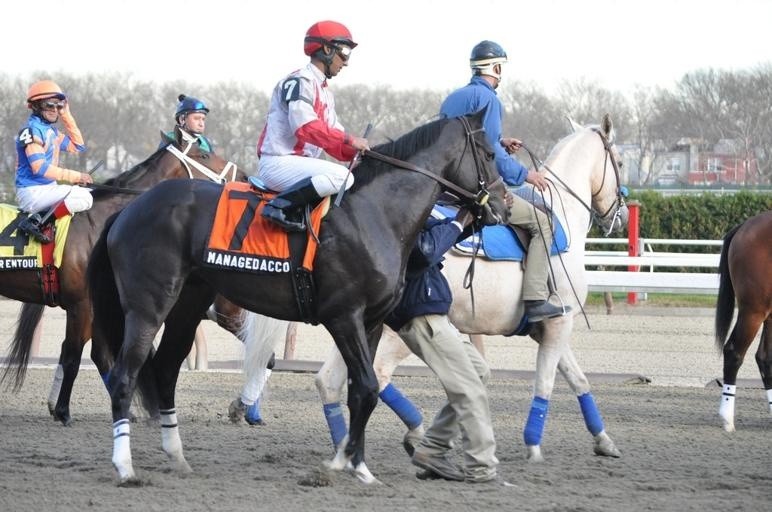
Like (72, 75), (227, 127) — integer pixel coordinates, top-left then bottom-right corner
(528, 300), (571, 323)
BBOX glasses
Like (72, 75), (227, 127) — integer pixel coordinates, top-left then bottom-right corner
(337, 47), (351, 61)
(42, 102), (65, 111)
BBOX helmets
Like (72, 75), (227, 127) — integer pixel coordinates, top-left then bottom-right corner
(28, 81), (66, 108)
(175, 95), (209, 119)
(304, 21), (357, 57)
(470, 41), (507, 66)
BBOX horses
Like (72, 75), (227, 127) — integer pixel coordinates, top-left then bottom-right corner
(83, 100), (513, 485)
(712, 210), (772, 434)
(0, 123), (248, 426)
(217, 111), (628, 464)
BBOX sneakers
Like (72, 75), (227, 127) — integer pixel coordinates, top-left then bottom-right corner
(412, 449), (465, 481)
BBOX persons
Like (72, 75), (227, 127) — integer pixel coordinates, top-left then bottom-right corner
(158, 92), (220, 155)
(15, 79), (95, 245)
(439, 39), (574, 323)
(257, 20), (371, 234)
(387, 193), (520, 490)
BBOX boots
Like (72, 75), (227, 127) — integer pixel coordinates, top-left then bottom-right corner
(261, 176), (322, 232)
(19, 206), (57, 243)
